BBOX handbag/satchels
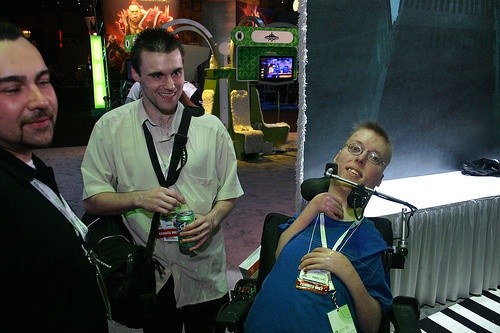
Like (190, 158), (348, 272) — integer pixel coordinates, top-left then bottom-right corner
(82, 210), (156, 329)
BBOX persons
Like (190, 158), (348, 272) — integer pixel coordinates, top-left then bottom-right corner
(80, 27), (244, 333)
(0, 30), (109, 333)
(240, 120), (393, 333)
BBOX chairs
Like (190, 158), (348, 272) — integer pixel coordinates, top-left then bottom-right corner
(201, 86), (290, 163)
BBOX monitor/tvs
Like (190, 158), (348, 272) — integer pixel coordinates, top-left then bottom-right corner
(259, 56), (294, 82)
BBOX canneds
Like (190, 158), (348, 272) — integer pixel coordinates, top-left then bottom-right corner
(176, 209), (197, 247)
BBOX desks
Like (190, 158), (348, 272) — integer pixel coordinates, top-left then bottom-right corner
(365, 170), (500, 307)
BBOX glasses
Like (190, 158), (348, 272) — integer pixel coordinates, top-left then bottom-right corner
(342, 144), (387, 168)
